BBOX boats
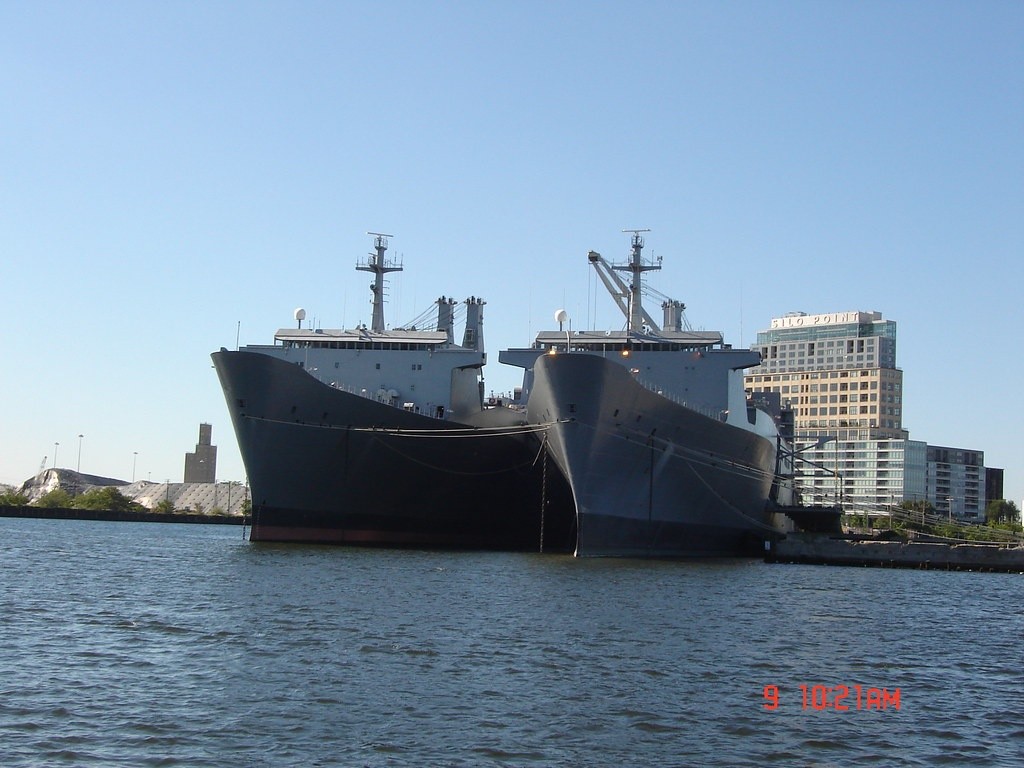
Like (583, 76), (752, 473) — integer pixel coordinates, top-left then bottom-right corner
(500, 229), (801, 558)
(207, 229), (577, 556)
